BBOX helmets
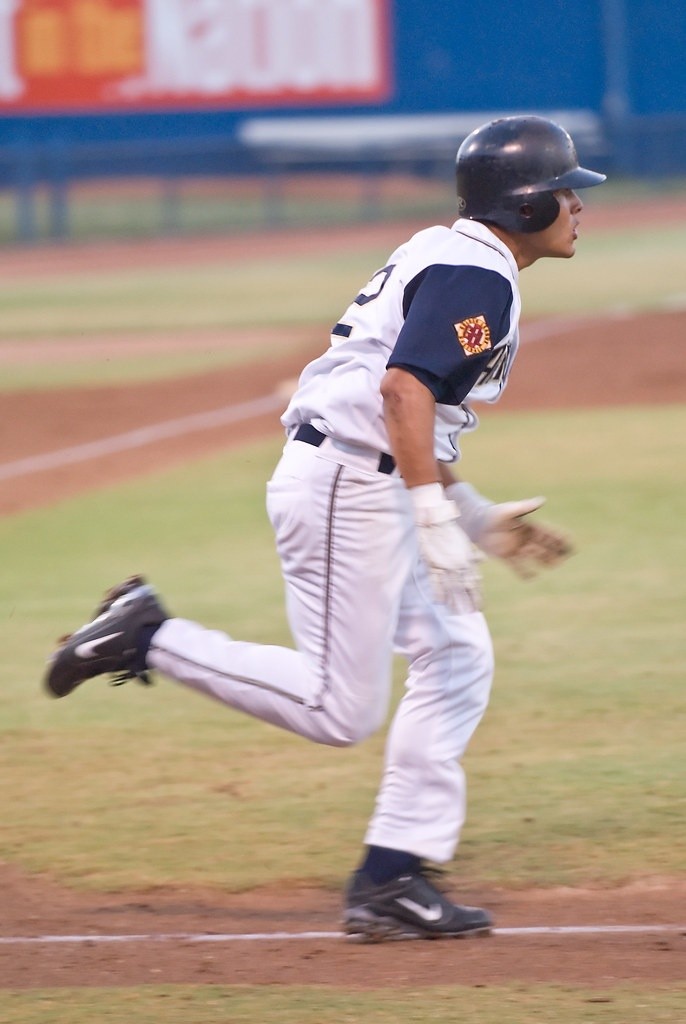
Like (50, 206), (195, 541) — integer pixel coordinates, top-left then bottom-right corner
(454, 115), (607, 234)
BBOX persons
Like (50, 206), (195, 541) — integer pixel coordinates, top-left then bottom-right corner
(39, 115), (609, 943)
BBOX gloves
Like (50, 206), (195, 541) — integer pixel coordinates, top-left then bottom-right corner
(445, 481), (574, 582)
(407, 480), (489, 614)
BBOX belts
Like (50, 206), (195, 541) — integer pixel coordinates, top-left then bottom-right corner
(294, 423), (396, 475)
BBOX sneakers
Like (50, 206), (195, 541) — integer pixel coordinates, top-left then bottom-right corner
(343, 858), (499, 942)
(43, 574), (169, 699)
